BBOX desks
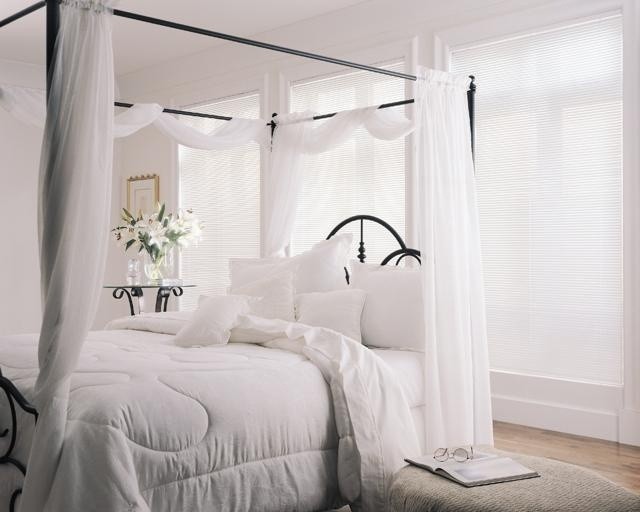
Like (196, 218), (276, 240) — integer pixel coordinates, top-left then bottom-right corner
(101, 281), (198, 315)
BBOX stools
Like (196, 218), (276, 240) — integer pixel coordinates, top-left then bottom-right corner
(390, 445), (640, 511)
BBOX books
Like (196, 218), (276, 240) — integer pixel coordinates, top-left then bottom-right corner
(404, 447), (541, 487)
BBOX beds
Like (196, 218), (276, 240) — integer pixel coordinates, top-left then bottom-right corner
(0, 212), (429, 512)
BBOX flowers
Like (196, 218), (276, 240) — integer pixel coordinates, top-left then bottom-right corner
(110, 201), (208, 265)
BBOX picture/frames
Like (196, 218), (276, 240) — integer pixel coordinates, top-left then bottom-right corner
(126, 174), (160, 232)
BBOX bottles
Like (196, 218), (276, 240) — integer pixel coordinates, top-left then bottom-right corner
(127, 258), (139, 278)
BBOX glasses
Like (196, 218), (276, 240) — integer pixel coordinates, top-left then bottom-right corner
(432, 446), (474, 463)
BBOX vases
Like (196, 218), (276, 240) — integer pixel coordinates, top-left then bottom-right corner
(142, 258), (165, 282)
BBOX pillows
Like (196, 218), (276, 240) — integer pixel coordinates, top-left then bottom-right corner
(171, 232), (424, 351)
